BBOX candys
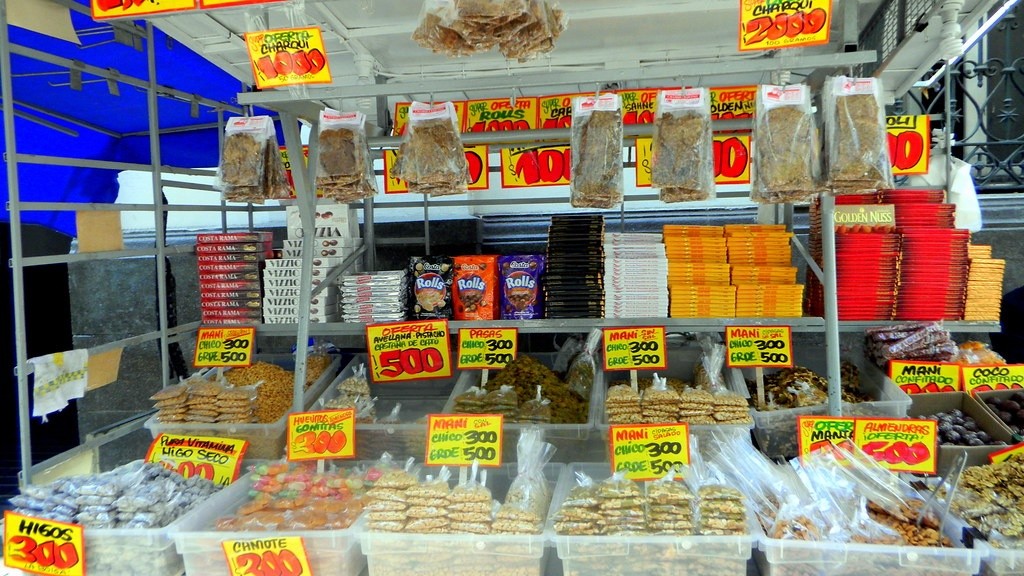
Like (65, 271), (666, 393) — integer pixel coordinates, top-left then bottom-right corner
(216, 461), (381, 532)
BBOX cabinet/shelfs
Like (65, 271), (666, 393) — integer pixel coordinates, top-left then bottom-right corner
(2, 1), (1024, 576)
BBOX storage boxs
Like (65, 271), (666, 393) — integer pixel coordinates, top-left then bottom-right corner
(459, 350), (604, 460)
(973, 386), (1024, 442)
(546, 461), (754, 576)
(355, 457), (552, 576)
(9, 458), (227, 576)
(139, 349), (340, 461)
(743, 460), (989, 576)
(168, 461), (372, 576)
(306, 352), (482, 464)
(730, 332), (915, 464)
(909, 389), (1022, 475)
(591, 339), (754, 462)
